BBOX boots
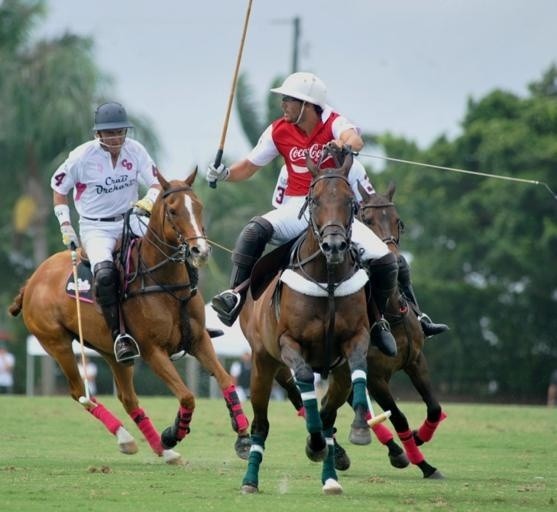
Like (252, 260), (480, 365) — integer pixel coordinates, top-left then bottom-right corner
(213, 264), (251, 327)
(366, 287), (398, 358)
(402, 285), (449, 336)
(100, 301), (136, 368)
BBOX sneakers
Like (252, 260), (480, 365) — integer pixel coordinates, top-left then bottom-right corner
(205, 328), (223, 338)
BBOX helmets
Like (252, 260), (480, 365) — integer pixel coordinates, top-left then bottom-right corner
(92, 102), (134, 131)
(269, 72), (327, 108)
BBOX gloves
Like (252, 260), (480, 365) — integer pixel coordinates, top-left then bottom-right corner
(205, 163), (230, 184)
(60, 225), (79, 250)
(134, 199), (152, 216)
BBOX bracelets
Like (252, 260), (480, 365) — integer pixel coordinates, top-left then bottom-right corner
(53, 204), (71, 225)
(144, 187), (161, 202)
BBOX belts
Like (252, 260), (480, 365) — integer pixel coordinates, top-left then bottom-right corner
(79, 212), (126, 222)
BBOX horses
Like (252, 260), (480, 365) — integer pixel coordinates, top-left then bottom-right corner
(237, 147), (375, 495)
(7, 161), (253, 466)
(300, 176), (448, 481)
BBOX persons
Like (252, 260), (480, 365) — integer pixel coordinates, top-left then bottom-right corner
(545, 369), (557, 404)
(78, 353), (98, 396)
(0, 346), (14, 395)
(271, 154), (450, 338)
(229, 350), (254, 405)
(205, 72), (401, 359)
(49, 101), (223, 366)
